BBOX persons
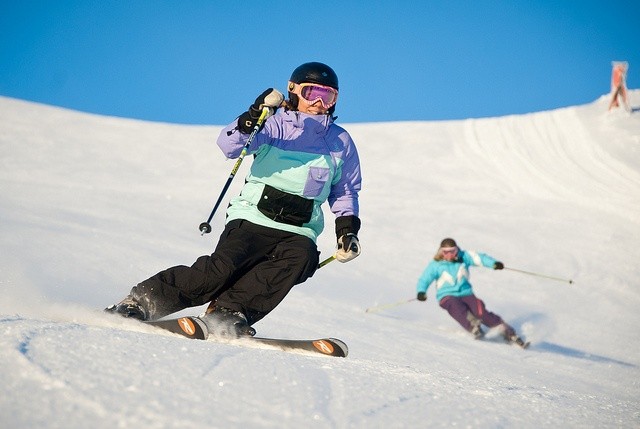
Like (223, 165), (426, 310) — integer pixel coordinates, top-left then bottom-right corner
(114, 60), (365, 337)
(415, 235), (532, 352)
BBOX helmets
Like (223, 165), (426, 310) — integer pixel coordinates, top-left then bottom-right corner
(440, 239), (458, 258)
(288, 62), (338, 114)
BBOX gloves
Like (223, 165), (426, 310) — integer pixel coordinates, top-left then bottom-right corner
(335, 215), (361, 263)
(238, 87), (283, 134)
(417, 292), (426, 301)
(494, 262), (504, 270)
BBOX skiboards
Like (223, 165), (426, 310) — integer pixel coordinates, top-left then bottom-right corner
(475, 335), (531, 349)
(142, 316), (348, 357)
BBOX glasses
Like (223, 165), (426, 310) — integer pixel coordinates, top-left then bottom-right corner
(287, 80), (338, 110)
(441, 247), (457, 254)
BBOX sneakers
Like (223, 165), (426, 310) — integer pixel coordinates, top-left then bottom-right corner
(116, 285), (157, 320)
(205, 299), (248, 327)
(505, 333), (519, 342)
(471, 325), (480, 335)
